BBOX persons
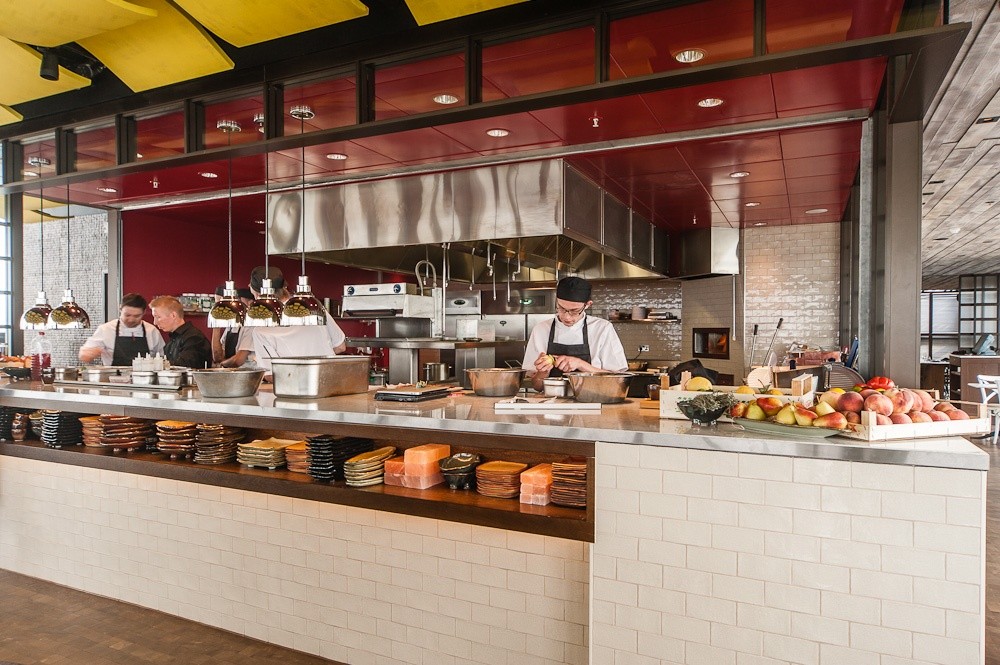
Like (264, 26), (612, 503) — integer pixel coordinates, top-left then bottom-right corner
(521, 277), (629, 392)
(212, 267), (346, 383)
(149, 295), (212, 369)
(78, 294), (165, 366)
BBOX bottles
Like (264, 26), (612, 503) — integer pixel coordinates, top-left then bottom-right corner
(132, 350), (170, 372)
(31, 332), (51, 381)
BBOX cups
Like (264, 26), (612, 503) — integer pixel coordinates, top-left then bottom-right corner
(632, 308), (645, 319)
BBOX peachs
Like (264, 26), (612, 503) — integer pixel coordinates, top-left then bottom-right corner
(819, 385), (971, 426)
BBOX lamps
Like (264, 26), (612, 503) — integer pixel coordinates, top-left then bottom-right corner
(243, 112), (283, 327)
(206, 120), (248, 327)
(280, 106), (326, 326)
(47, 183), (90, 329)
(19, 156), (53, 330)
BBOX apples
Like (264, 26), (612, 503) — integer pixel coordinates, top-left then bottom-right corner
(684, 376), (782, 395)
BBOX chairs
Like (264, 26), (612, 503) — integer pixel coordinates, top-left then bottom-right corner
(978, 374), (1000, 444)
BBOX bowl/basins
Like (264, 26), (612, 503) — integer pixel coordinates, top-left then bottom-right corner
(461, 367), (526, 397)
(3, 367), (30, 377)
(190, 367), (267, 397)
(14, 412), (29, 422)
(11, 428), (26, 441)
(629, 363), (647, 371)
(677, 400), (728, 423)
(12, 420), (28, 429)
(562, 372), (637, 405)
(40, 368), (56, 384)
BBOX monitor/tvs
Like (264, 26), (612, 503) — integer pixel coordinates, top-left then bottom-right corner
(973, 334), (995, 353)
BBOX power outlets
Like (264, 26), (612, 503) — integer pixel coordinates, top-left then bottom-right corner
(638, 344), (649, 351)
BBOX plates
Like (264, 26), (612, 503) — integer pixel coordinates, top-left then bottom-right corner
(0, 406), (589, 513)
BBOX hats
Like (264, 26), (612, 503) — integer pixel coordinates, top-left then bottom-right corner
(556, 277), (593, 302)
(250, 265), (284, 294)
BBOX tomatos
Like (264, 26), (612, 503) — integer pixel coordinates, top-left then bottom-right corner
(829, 377), (895, 393)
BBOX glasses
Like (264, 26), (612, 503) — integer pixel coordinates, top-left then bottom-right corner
(556, 299), (586, 316)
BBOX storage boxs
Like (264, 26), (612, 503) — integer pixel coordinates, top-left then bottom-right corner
(658, 384), (814, 422)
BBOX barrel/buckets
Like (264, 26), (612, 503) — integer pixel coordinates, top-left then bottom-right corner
(422, 362), (452, 382)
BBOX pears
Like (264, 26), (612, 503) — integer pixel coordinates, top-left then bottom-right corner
(724, 395), (848, 430)
